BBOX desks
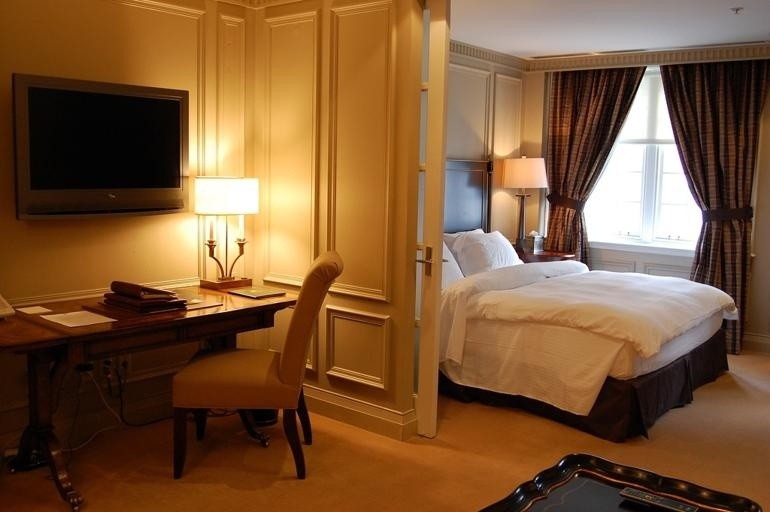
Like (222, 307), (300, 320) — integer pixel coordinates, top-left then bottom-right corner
(0, 278), (297, 512)
(478, 452), (762, 512)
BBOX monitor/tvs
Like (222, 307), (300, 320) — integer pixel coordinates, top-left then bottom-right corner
(10, 71), (191, 223)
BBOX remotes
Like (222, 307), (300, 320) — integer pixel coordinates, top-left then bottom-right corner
(618, 487), (699, 512)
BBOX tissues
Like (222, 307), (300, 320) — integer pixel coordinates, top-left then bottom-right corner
(526, 230), (542, 253)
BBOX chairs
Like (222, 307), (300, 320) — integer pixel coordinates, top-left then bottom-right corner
(172, 249), (344, 478)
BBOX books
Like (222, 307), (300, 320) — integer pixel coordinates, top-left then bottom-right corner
(97, 281), (189, 313)
(227, 285), (286, 300)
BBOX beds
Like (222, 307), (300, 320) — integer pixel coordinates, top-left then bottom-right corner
(437, 230), (738, 441)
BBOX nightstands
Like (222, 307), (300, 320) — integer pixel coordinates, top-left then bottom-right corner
(517, 251), (576, 264)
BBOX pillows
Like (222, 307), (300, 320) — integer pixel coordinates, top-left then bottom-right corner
(443, 228), (484, 249)
(453, 231), (525, 277)
(441, 240), (464, 288)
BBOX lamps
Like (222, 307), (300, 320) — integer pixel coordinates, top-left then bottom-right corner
(500, 156), (548, 252)
(194, 176), (259, 291)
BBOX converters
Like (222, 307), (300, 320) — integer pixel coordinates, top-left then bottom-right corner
(75, 361), (94, 373)
